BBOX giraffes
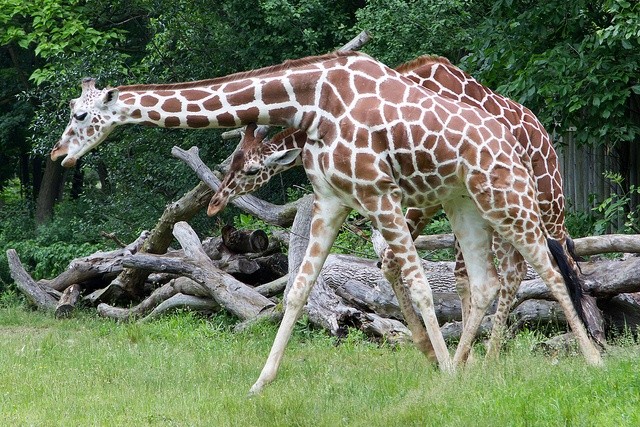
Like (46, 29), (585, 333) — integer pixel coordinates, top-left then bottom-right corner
(51, 50), (607, 394)
(208, 57), (587, 375)
